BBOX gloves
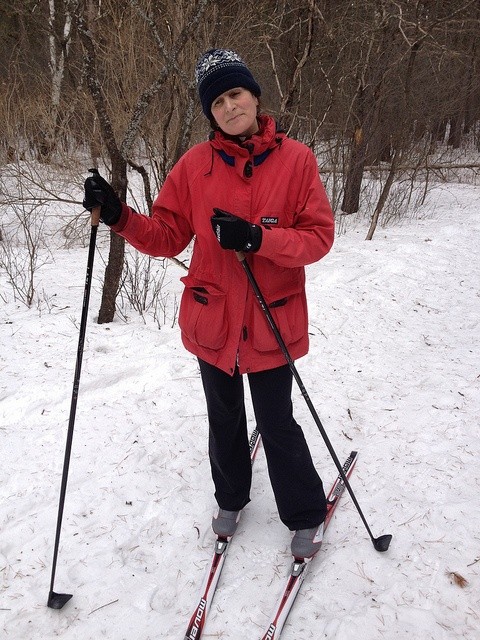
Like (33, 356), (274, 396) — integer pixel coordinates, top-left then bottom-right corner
(83, 168), (122, 226)
(210, 208), (262, 253)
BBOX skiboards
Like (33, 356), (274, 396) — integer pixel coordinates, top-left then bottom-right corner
(182, 397), (358, 638)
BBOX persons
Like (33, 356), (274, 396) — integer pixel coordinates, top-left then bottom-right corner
(82, 50), (335, 560)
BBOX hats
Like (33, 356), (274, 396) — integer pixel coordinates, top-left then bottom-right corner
(199, 49), (261, 128)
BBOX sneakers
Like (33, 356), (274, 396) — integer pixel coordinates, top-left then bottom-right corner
(291, 522), (324, 560)
(212, 503), (242, 537)
